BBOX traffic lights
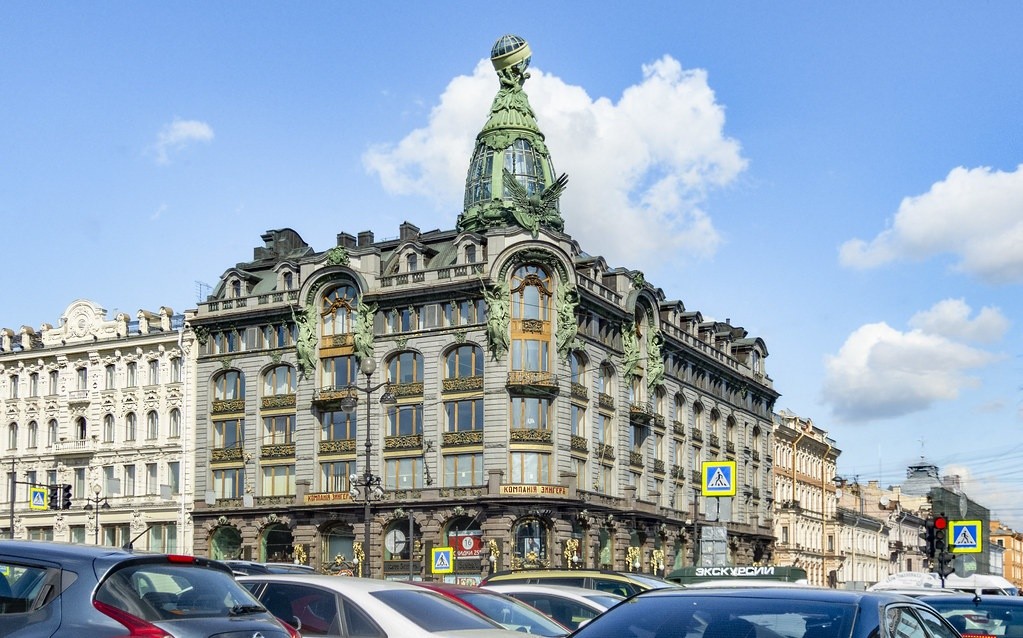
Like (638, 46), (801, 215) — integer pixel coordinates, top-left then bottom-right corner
(939, 553), (956, 578)
(933, 517), (948, 549)
(918, 520), (933, 554)
(62, 484), (72, 510)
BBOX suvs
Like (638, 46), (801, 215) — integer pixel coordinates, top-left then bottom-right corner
(217, 560), (323, 576)
(479, 567), (686, 599)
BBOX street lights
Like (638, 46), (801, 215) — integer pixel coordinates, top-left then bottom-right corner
(341, 356), (397, 578)
(83, 484), (111, 544)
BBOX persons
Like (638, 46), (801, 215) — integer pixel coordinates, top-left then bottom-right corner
(497, 70), (531, 94)
(349, 302), (369, 352)
(528, 538), (539, 551)
(292, 314), (313, 359)
(1018, 588), (1023, 596)
(561, 294), (581, 348)
(554, 605), (578, 630)
(483, 292), (509, 346)
(650, 337), (665, 382)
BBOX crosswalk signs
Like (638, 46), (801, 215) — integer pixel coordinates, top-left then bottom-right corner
(702, 461), (737, 496)
(948, 519), (984, 553)
(431, 547), (454, 573)
(30, 488), (47, 510)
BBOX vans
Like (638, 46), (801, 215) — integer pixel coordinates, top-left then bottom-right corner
(930, 574), (1019, 596)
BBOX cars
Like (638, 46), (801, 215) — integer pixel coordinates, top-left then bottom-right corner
(916, 596), (1023, 638)
(0, 539), (303, 638)
(397, 580), (574, 638)
(480, 585), (627, 633)
(165, 574), (550, 638)
(563, 586), (962, 638)
